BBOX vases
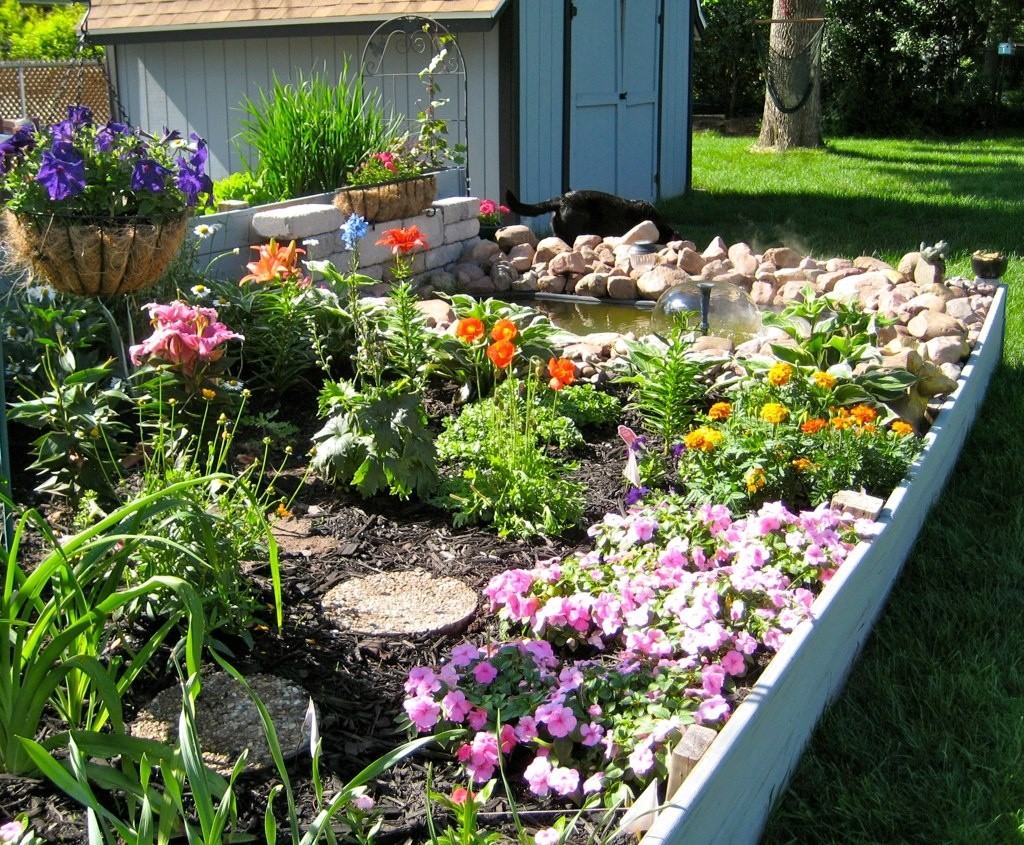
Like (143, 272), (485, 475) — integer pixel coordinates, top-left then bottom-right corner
(7, 208), (192, 297)
(334, 174), (438, 226)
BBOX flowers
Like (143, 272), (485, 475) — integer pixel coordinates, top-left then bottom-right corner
(2, 104), (215, 205)
(346, 153), (420, 187)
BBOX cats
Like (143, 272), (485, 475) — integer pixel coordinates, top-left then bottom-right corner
(505, 188), (684, 246)
